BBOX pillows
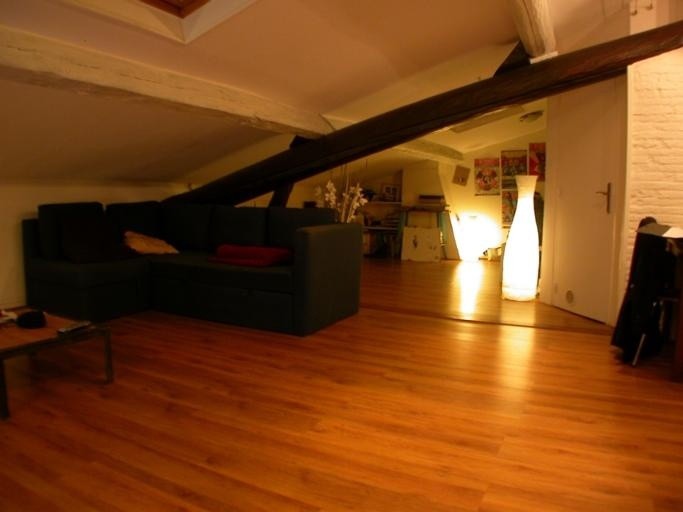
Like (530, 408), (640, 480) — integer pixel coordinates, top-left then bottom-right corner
(123, 231), (179, 256)
(215, 242), (289, 267)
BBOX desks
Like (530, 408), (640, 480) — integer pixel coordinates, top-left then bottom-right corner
(635, 223), (683, 243)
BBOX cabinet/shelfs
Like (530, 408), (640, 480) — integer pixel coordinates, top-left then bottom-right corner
(363, 200), (401, 231)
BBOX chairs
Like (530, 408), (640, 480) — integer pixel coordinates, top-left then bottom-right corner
(635, 217), (678, 366)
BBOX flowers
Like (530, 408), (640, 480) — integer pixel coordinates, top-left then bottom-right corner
(314, 179), (368, 223)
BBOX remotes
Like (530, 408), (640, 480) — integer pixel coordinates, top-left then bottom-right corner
(57, 320), (91, 335)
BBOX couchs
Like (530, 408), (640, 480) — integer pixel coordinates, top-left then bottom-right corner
(22, 197), (364, 338)
